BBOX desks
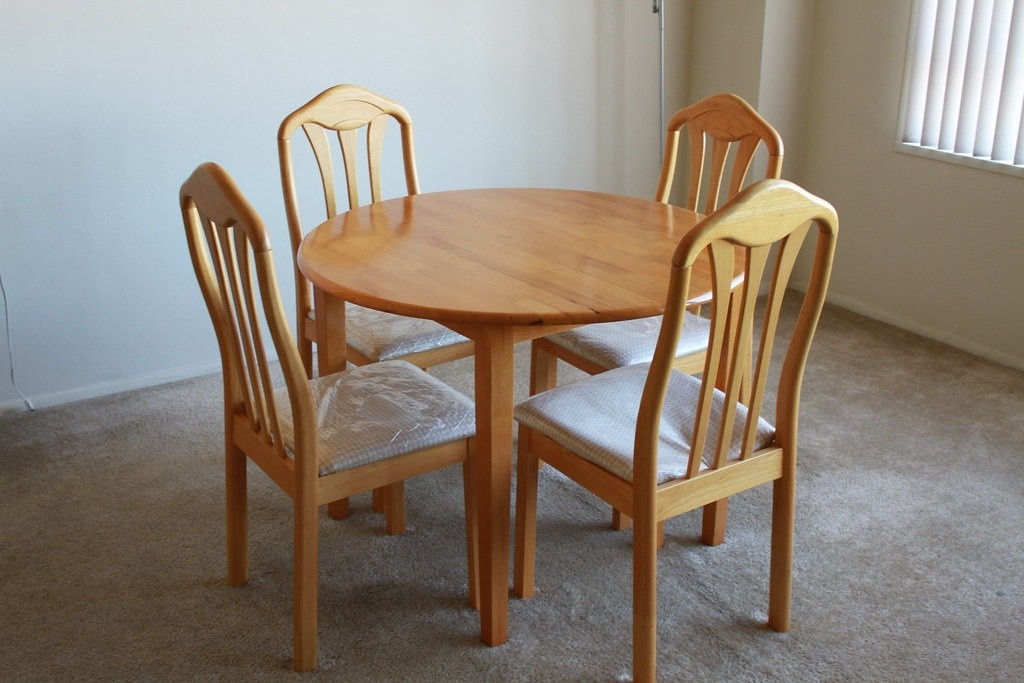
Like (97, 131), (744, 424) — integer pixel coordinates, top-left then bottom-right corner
(295, 188), (754, 646)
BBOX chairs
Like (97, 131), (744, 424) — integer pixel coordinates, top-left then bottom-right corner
(275, 85), (473, 535)
(179, 164), (478, 673)
(510, 179), (852, 683)
(527, 94), (782, 531)
